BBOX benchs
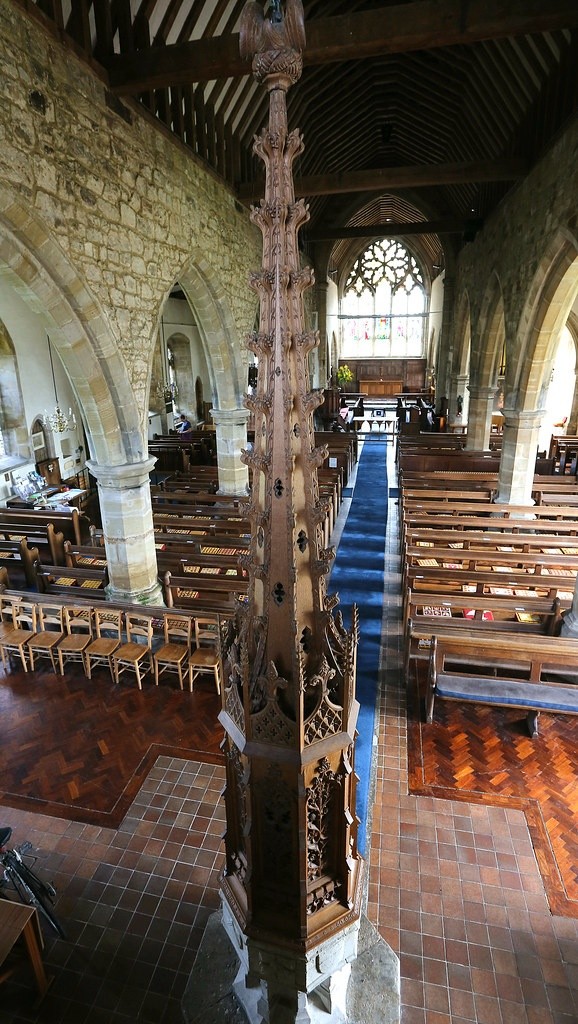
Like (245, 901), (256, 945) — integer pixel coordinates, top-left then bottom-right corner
(397, 431), (578, 740)
(0, 428), (359, 620)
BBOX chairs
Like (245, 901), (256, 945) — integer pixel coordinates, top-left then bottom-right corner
(57, 604), (93, 676)
(27, 602), (66, 674)
(0, 595), (22, 659)
(189, 617), (222, 695)
(113, 612), (156, 690)
(84, 607), (124, 682)
(0, 602), (36, 672)
(153, 612), (192, 689)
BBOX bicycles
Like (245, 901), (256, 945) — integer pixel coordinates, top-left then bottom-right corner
(0, 826), (67, 941)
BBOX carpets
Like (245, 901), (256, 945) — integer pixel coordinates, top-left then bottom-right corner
(327, 431), (389, 858)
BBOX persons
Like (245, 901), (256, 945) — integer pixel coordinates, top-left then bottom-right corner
(177, 414), (192, 434)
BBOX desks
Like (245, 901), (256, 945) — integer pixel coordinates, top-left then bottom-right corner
(34, 489), (89, 519)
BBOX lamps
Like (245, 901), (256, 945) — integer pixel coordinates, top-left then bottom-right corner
(154, 315), (179, 402)
(43, 335), (78, 434)
